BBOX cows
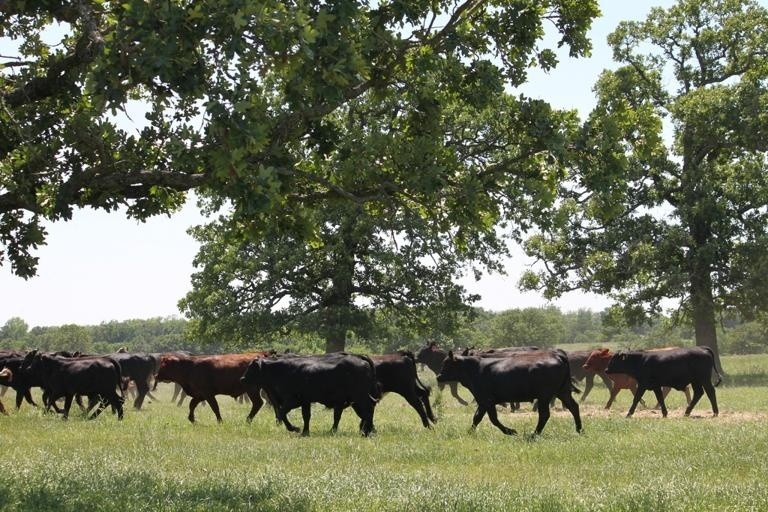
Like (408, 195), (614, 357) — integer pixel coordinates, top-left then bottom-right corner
(0, 340), (723, 440)
